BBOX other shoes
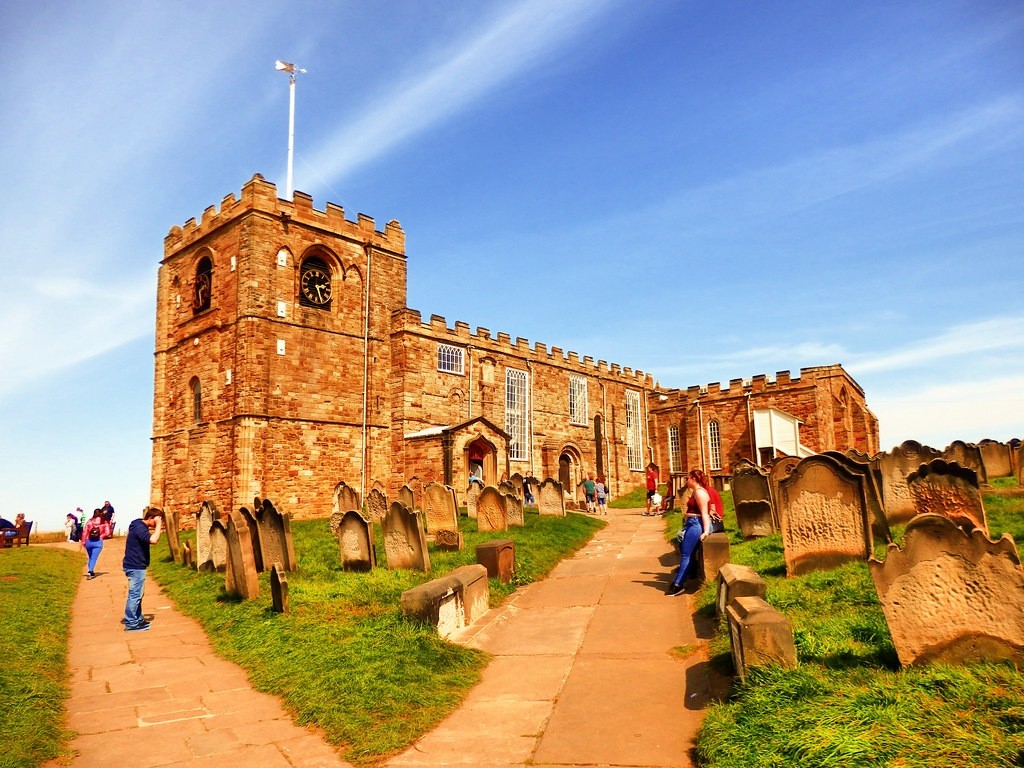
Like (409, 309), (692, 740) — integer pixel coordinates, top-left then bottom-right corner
(124, 621), (150, 633)
(666, 583), (686, 597)
(87, 572), (95, 580)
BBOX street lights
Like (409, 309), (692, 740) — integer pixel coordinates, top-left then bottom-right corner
(274, 59), (307, 204)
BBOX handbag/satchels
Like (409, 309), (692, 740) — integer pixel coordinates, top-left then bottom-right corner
(651, 493), (662, 507)
(710, 510), (726, 533)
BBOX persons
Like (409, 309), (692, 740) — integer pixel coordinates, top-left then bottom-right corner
(0, 515), (16, 536)
(678, 486), (724, 577)
(665, 469), (711, 596)
(523, 471), (535, 509)
(642, 462), (662, 517)
(592, 477), (609, 516)
(64, 507), (86, 544)
(122, 507), (164, 632)
(99, 501), (114, 540)
(14, 513), (25, 535)
(582, 475), (597, 513)
(82, 509), (109, 580)
(468, 471), (485, 490)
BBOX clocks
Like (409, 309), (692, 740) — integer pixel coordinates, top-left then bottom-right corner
(301, 269), (333, 305)
(191, 274), (210, 309)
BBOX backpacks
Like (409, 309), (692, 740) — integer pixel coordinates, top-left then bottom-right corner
(89, 521), (103, 541)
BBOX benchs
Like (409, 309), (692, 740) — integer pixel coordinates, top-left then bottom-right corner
(3, 520), (33, 547)
